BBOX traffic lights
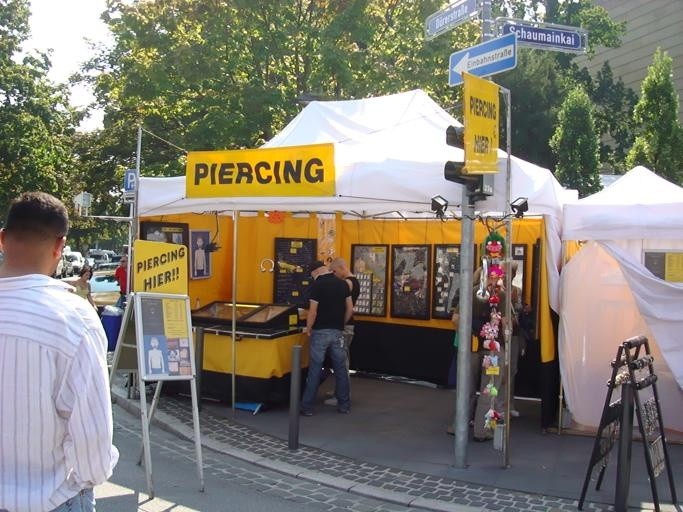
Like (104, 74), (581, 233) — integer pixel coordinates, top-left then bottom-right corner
(444, 125), (494, 197)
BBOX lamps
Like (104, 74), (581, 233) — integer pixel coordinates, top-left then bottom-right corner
(509, 196), (528, 219)
(430, 195), (448, 218)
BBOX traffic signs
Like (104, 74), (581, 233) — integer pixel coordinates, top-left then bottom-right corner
(447, 31), (518, 86)
(498, 15), (583, 49)
(428, 0), (477, 37)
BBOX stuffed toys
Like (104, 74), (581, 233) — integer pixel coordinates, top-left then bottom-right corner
(480, 232), (508, 433)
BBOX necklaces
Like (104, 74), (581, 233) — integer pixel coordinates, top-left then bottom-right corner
(591, 397), (665, 483)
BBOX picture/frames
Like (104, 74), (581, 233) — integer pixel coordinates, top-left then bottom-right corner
(139, 221), (211, 281)
(349, 242), (527, 320)
(271, 236), (317, 310)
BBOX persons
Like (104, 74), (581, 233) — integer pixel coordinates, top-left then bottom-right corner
(114, 255), (131, 295)
(168, 349), (177, 361)
(79, 259), (93, 280)
(299, 259), (352, 416)
(323, 259), (359, 405)
(104, 253), (109, 263)
(1, 190), (123, 512)
(64, 268), (97, 310)
(193, 237), (207, 276)
(146, 336), (166, 373)
(445, 260), (520, 443)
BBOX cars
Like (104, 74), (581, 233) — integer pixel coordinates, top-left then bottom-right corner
(48, 244), (124, 316)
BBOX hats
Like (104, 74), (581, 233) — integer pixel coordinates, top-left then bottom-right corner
(305, 261), (324, 276)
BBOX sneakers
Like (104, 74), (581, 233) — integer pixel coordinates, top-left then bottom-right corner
(474, 431), (494, 441)
(323, 399), (338, 406)
(325, 392), (335, 398)
(447, 426), (455, 435)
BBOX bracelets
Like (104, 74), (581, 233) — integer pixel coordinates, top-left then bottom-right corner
(611, 334), (657, 387)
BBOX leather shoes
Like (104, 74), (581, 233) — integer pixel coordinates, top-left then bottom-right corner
(511, 410), (520, 418)
